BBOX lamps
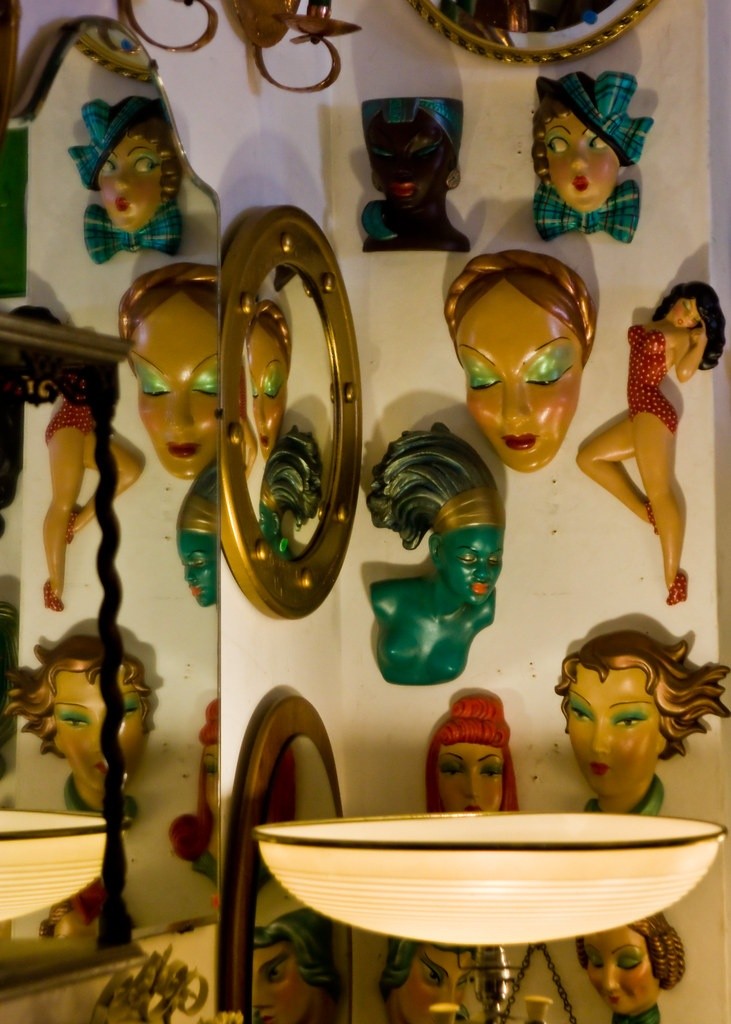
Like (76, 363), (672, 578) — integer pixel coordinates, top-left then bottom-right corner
(0, 805), (133, 928)
(249, 814), (727, 1024)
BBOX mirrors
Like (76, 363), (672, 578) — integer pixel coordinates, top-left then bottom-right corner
(219, 204), (365, 619)
(406, 0), (661, 65)
(0, 13), (223, 996)
(212, 684), (354, 1024)
